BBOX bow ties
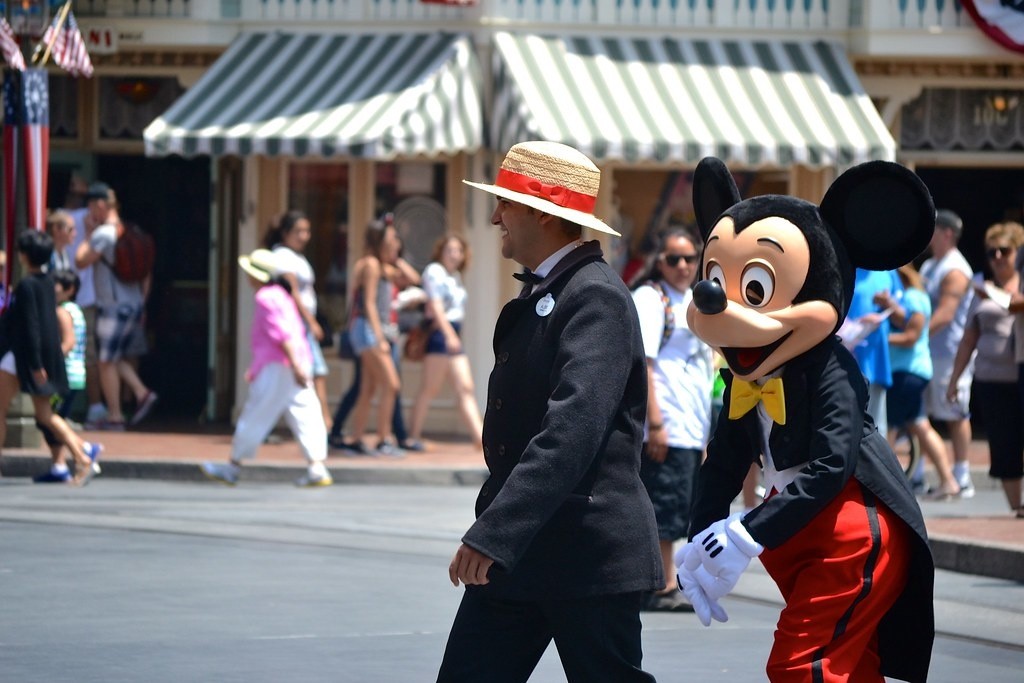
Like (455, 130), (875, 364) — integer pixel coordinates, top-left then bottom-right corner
(513, 272), (543, 284)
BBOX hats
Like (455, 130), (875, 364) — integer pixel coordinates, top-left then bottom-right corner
(77, 184), (109, 207)
(462, 140), (622, 237)
(236, 249), (284, 284)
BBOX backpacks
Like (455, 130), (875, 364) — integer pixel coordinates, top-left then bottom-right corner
(98, 222), (156, 285)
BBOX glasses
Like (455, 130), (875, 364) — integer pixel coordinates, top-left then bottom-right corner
(657, 254), (699, 267)
(983, 246), (1012, 258)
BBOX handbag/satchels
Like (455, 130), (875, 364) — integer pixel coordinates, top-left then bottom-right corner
(404, 326), (426, 362)
(337, 328), (352, 358)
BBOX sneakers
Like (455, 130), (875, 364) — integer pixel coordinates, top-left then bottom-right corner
(957, 478), (975, 497)
(33, 467), (73, 484)
(650, 586), (694, 612)
(199, 460), (240, 484)
(88, 442), (104, 460)
(293, 467), (333, 486)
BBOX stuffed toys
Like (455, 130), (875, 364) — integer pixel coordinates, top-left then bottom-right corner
(676, 158), (935, 683)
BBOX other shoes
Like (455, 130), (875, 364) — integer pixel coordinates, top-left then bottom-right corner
(350, 438), (370, 457)
(375, 438), (401, 455)
(66, 456), (100, 486)
(130, 390), (156, 422)
(96, 418), (123, 430)
(327, 434), (346, 447)
(400, 438), (427, 450)
(909, 480), (930, 493)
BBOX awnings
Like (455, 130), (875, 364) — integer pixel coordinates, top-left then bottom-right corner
(143, 26), (897, 173)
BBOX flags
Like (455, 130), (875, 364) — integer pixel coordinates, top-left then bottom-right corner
(36, 1), (95, 79)
(0, 5), (26, 73)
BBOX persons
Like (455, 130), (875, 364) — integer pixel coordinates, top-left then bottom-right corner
(199, 208), (336, 488)
(434, 142), (665, 681)
(326, 220), (482, 453)
(839, 214), (1024, 518)
(0, 181), (158, 489)
(628, 228), (770, 612)
